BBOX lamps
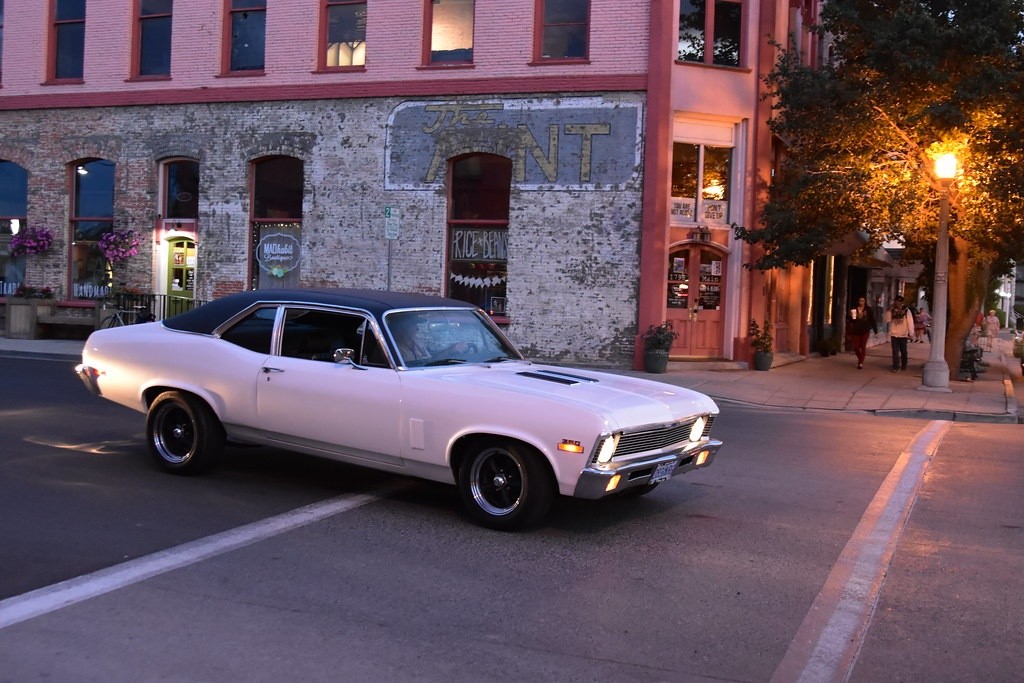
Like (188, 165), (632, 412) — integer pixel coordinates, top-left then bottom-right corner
(693, 226), (712, 243)
(168, 220), (182, 231)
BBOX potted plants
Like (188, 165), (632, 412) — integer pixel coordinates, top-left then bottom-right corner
(750, 318), (774, 371)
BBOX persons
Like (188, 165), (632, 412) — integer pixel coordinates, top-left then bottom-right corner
(884, 295), (932, 373)
(968, 309), (1001, 352)
(848, 297), (880, 369)
(396, 318), (469, 363)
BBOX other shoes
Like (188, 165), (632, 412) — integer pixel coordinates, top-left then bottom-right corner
(857, 362), (862, 369)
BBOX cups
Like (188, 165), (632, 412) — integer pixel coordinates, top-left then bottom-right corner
(851, 310), (856, 319)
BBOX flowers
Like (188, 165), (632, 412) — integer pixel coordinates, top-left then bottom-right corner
(15, 284), (53, 298)
(9, 226), (53, 259)
(95, 228), (139, 265)
(641, 321), (679, 350)
(112, 284), (140, 296)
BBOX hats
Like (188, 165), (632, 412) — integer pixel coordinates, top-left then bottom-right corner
(892, 296), (904, 302)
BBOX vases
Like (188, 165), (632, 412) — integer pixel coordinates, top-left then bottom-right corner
(99, 295), (145, 329)
(5, 298), (54, 339)
(644, 349), (668, 373)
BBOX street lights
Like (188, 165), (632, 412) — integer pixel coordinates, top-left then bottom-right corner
(919, 143), (960, 392)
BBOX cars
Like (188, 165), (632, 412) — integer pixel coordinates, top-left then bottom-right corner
(73, 290), (728, 527)
(1010, 317), (1024, 374)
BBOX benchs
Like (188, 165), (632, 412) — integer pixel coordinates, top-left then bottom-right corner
(925, 328), (980, 378)
(40, 298), (99, 328)
(0, 297), (9, 330)
(230, 326), (367, 361)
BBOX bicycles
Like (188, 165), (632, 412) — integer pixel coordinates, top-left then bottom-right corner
(920, 320), (932, 343)
(99, 302), (156, 331)
